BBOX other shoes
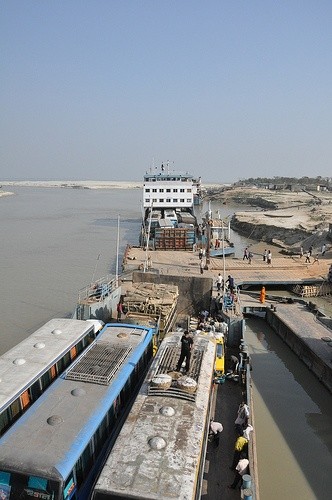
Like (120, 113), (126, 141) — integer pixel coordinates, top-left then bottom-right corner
(228, 485), (232, 488)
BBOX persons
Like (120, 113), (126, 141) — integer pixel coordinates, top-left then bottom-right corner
(227, 355), (239, 374)
(174, 331), (193, 372)
(199, 259), (205, 274)
(306, 251), (311, 263)
(217, 239), (220, 247)
(322, 244), (327, 256)
(213, 293), (223, 315)
(234, 401), (249, 435)
(259, 285), (266, 303)
(267, 250), (271, 263)
(216, 274), (222, 291)
(225, 275), (234, 289)
(199, 247), (203, 259)
(243, 248), (248, 260)
(117, 302), (122, 318)
(328, 265), (332, 281)
(197, 309), (223, 332)
(263, 249), (266, 261)
(209, 422), (224, 447)
(312, 254), (320, 265)
(309, 245), (313, 256)
(248, 252), (253, 264)
(300, 246), (305, 258)
(231, 452), (249, 488)
(230, 423), (253, 468)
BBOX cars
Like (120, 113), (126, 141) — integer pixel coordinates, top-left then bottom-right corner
(198, 331), (226, 384)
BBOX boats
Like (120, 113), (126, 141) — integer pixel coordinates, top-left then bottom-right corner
(198, 209), (236, 258)
(139, 161), (203, 251)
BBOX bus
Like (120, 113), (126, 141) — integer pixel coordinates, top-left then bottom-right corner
(0, 317), (105, 436)
(89, 331), (220, 499)
(0, 321), (157, 500)
